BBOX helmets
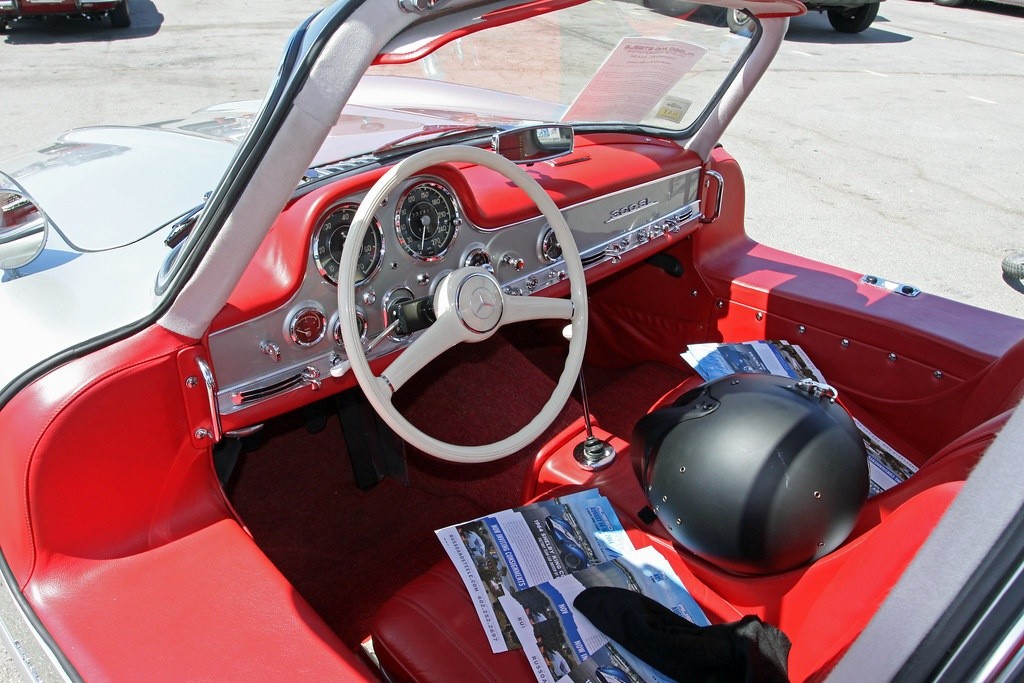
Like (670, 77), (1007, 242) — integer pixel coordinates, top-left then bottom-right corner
(627, 371), (870, 576)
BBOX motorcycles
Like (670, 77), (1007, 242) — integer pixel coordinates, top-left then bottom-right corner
(641, 1), (887, 37)
(0, 0), (1024, 682)
(0, 0), (131, 31)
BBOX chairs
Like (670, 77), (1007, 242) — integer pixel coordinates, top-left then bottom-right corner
(371, 408), (1017, 683)
(646, 336), (1024, 498)
